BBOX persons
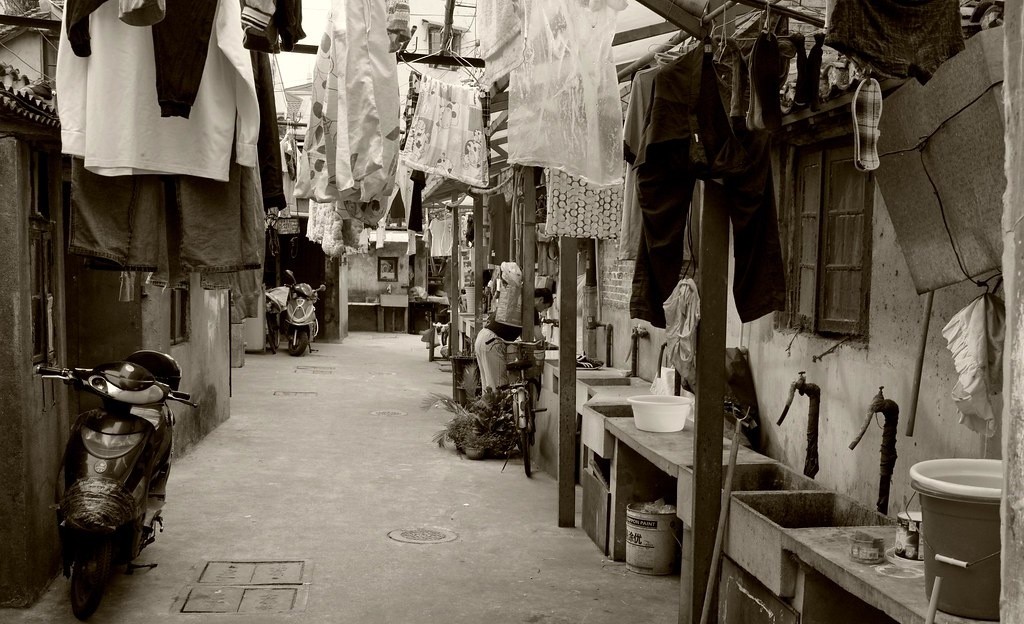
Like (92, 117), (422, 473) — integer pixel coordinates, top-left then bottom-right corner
(474, 287), (553, 417)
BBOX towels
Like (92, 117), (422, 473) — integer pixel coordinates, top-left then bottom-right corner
(544, 167), (624, 240)
(480, 0), (532, 86)
(398, 73), (490, 188)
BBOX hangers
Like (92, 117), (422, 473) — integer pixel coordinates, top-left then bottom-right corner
(397, 27), (483, 89)
(649, 0), (825, 67)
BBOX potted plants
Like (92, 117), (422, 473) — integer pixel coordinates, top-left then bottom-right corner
(457, 381), (480, 408)
(421, 366), (499, 460)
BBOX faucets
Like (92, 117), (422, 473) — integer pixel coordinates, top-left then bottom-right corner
(386, 284), (392, 293)
(656, 341), (669, 378)
(776, 371), (807, 428)
(586, 314), (598, 331)
(848, 386), (887, 451)
(538, 315), (548, 328)
(625, 326), (638, 363)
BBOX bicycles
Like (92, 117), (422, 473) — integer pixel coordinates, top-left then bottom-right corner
(265, 301), (281, 355)
(484, 336), (549, 479)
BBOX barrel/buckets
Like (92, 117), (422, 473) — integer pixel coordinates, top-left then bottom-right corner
(903, 480), (1001, 621)
(626, 501), (682, 576)
(894, 510), (925, 564)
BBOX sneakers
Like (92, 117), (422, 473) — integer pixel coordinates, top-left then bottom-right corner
(576, 355), (604, 371)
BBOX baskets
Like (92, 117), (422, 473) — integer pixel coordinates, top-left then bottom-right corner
(502, 339), (546, 373)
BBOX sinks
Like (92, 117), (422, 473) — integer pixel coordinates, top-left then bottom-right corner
(380, 294), (409, 307)
(466, 319), (488, 339)
(510, 341), (560, 366)
(458, 313), (475, 332)
(720, 488), (902, 598)
(583, 403), (635, 458)
(543, 359), (607, 393)
(677, 460), (831, 529)
(576, 377), (654, 416)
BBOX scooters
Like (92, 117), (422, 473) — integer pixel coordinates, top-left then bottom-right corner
(33, 349), (198, 619)
(283, 270), (326, 357)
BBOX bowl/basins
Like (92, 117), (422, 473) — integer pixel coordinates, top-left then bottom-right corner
(626, 395), (691, 432)
(909, 457), (1003, 498)
